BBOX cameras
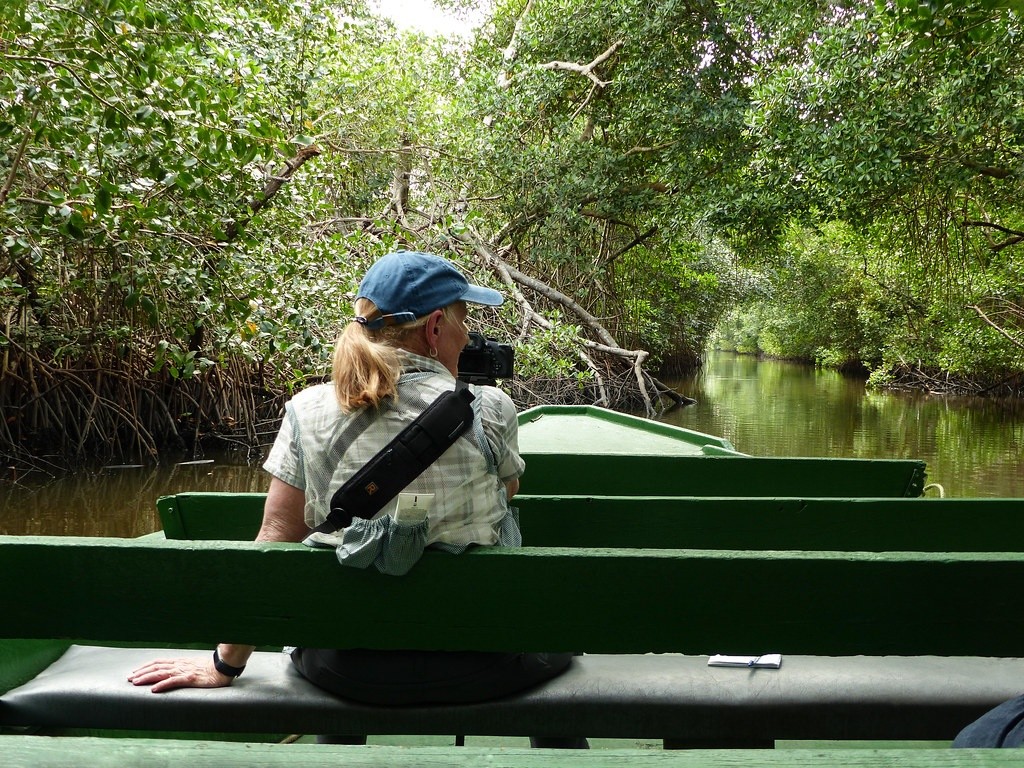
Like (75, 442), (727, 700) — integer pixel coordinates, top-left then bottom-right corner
(455, 331), (515, 387)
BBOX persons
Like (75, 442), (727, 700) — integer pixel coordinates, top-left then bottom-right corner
(128, 249), (592, 752)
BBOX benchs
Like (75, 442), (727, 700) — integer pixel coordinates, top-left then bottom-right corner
(0, 456), (1024, 768)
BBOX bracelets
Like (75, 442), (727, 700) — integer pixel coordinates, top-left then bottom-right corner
(213, 647), (245, 678)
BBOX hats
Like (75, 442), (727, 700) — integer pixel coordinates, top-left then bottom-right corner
(352, 249), (505, 329)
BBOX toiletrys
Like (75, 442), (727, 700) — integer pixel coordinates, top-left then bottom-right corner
(394, 492), (435, 529)
